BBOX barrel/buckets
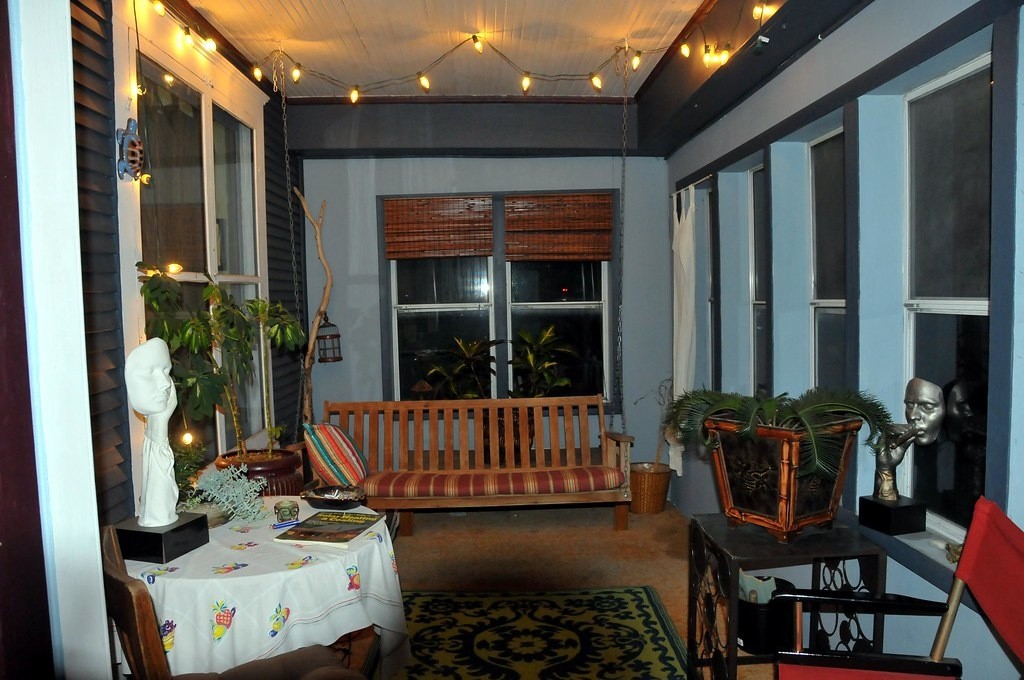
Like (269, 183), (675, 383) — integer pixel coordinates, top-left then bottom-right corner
(737, 576), (797, 653)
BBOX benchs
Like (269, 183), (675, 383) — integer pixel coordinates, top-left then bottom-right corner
(324, 394), (635, 537)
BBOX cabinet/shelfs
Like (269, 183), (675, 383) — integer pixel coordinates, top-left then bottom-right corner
(687, 506), (888, 680)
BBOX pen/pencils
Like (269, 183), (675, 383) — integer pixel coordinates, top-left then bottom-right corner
(271, 519), (300, 530)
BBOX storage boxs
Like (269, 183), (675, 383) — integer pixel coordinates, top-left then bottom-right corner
(115, 513), (210, 564)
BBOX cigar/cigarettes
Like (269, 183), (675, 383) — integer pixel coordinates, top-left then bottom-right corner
(889, 427), (919, 449)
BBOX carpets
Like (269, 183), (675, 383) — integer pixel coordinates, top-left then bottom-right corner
(356, 584), (687, 680)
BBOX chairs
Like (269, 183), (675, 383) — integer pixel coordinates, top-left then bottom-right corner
(776, 495), (1024, 680)
(100, 524), (342, 679)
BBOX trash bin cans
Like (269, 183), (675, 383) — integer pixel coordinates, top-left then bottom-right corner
(629, 461), (671, 515)
(735, 576), (797, 655)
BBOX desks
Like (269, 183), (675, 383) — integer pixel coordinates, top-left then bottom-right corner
(113, 496), (415, 674)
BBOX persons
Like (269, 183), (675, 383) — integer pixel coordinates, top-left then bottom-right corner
(872, 377), (946, 501)
(124, 337), (180, 528)
(943, 379), (987, 444)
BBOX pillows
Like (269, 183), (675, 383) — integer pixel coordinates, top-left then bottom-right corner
(303, 420), (370, 486)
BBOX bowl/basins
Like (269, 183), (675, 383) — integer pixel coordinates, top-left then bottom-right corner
(300, 485), (365, 510)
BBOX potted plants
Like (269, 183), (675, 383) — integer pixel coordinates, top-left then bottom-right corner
(172, 443), (236, 528)
(417, 327), (575, 462)
(135, 261), (306, 499)
(662, 385), (898, 546)
(629, 377), (675, 514)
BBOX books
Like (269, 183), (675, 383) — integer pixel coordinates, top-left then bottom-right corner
(274, 511), (387, 548)
